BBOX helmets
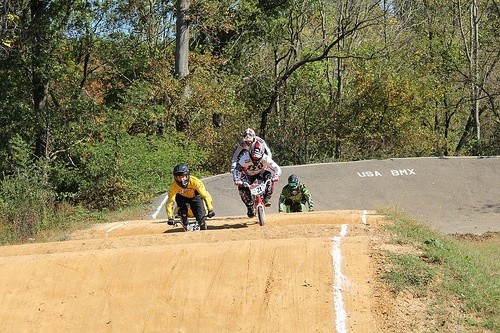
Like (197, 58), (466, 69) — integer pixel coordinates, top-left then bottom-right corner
(173, 163), (190, 188)
(243, 127), (256, 142)
(249, 140), (264, 157)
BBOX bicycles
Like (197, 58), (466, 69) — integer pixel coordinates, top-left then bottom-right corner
(168, 213), (209, 232)
(238, 177), (277, 226)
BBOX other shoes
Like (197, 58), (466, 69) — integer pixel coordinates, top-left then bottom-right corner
(247, 206), (255, 217)
(201, 221), (207, 229)
(262, 198), (272, 207)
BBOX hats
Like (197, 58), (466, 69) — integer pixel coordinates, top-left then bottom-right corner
(288, 173), (299, 186)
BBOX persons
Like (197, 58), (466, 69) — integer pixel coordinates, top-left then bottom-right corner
(230, 128), (282, 218)
(166, 164), (213, 224)
(278, 174), (315, 213)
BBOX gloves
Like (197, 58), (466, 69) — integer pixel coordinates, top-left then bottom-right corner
(272, 176), (279, 181)
(207, 210), (215, 217)
(235, 179), (242, 185)
(309, 206), (314, 211)
(167, 218), (175, 224)
